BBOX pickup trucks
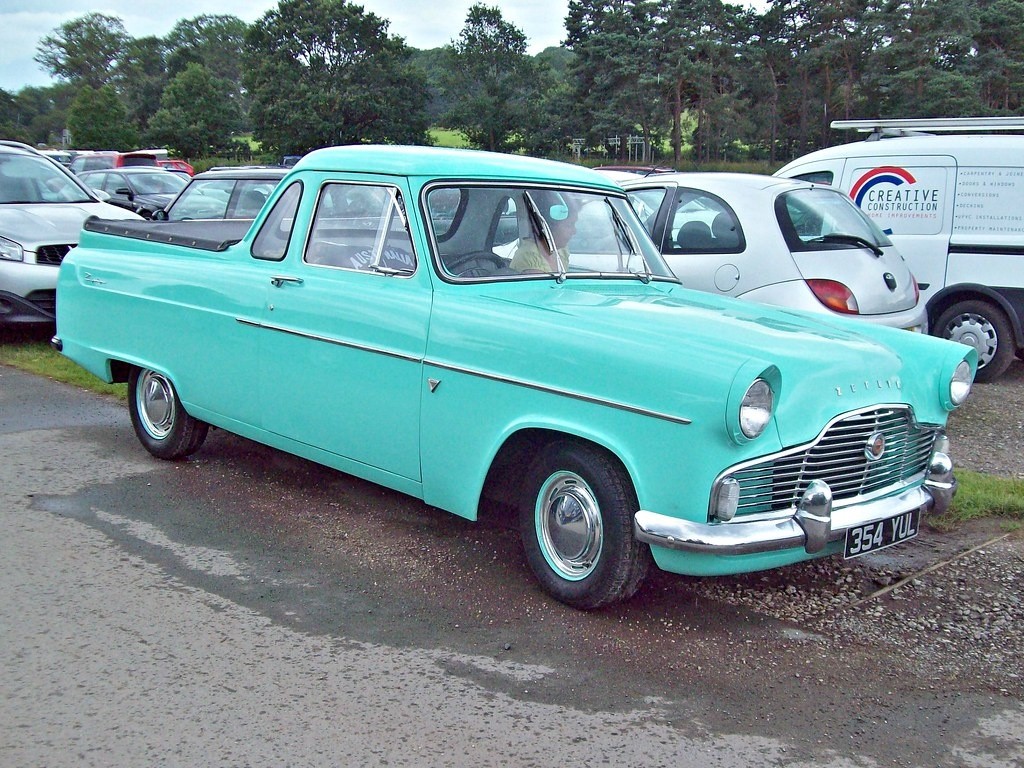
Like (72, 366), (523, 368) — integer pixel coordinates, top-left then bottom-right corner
(53, 145), (980, 611)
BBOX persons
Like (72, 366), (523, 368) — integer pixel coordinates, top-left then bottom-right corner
(507, 203), (578, 274)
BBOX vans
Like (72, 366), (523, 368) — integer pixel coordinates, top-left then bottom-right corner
(771, 115), (1024, 382)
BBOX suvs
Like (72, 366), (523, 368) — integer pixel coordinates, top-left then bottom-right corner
(283, 156), (303, 170)
(593, 165), (677, 177)
(0, 139), (146, 329)
(39, 149), (74, 172)
(46, 166), (189, 220)
(151, 165), (406, 220)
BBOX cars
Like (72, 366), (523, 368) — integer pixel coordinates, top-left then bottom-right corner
(612, 172), (929, 339)
(68, 151), (161, 174)
(157, 159), (195, 177)
